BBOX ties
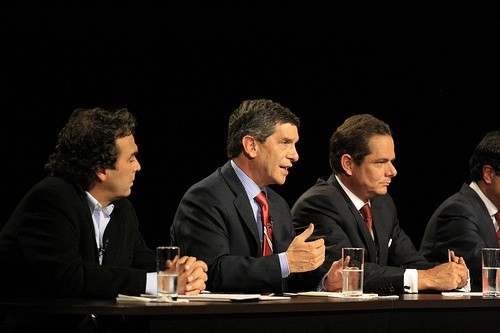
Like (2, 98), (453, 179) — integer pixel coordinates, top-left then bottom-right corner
(254, 191), (273, 256)
(360, 204), (372, 231)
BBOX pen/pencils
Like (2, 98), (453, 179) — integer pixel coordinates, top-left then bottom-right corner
(447, 249), (451, 263)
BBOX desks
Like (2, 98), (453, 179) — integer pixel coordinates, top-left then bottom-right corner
(0, 289), (500, 333)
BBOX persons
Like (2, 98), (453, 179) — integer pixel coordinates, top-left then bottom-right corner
(169, 99), (359, 294)
(290, 114), (468, 296)
(419, 131), (500, 292)
(0, 108), (208, 333)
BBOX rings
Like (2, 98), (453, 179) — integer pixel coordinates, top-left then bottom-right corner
(467, 278), (470, 282)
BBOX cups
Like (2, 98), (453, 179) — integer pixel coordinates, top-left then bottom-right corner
(155, 247), (180, 302)
(341, 247), (365, 298)
(481, 248), (500, 299)
